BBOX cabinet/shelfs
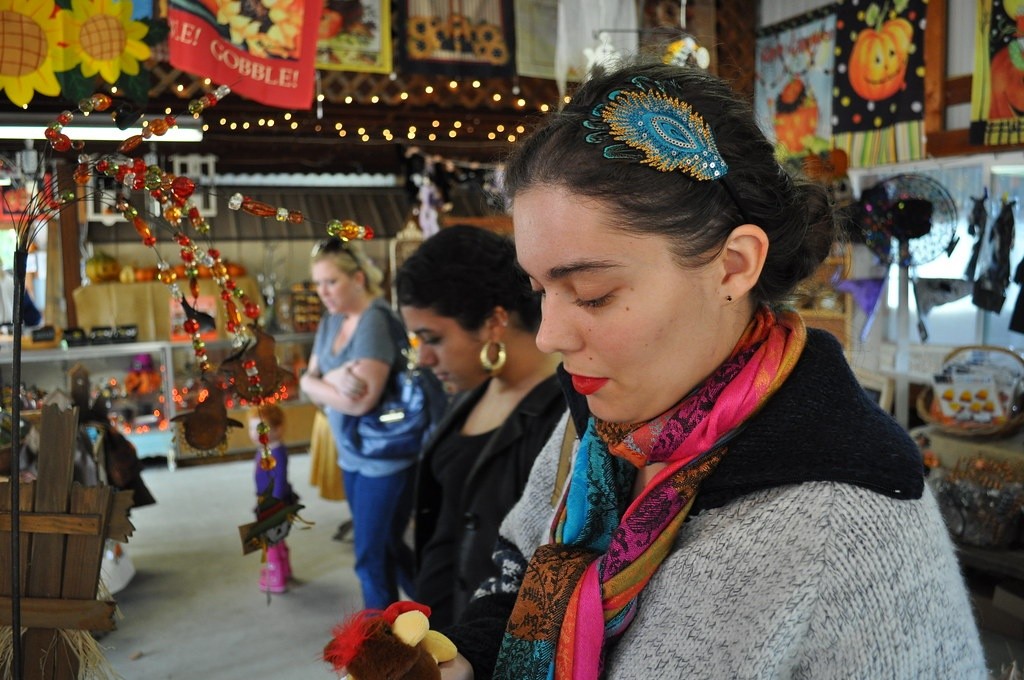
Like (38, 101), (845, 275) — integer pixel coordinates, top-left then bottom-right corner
(795, 237), (851, 357)
(0, 327), (318, 471)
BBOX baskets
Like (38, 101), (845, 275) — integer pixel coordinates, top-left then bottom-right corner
(917, 345), (1024, 437)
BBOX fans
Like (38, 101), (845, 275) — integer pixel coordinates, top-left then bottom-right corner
(858, 174), (958, 432)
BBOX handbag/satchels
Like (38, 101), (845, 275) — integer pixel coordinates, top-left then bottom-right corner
(342, 305), (427, 459)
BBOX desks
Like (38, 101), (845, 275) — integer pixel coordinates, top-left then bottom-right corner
(906, 424), (1024, 490)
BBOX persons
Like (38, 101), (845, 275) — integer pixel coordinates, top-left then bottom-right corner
(297, 239), (425, 611)
(337, 61), (989, 680)
(246, 405), (297, 592)
(390, 225), (581, 637)
